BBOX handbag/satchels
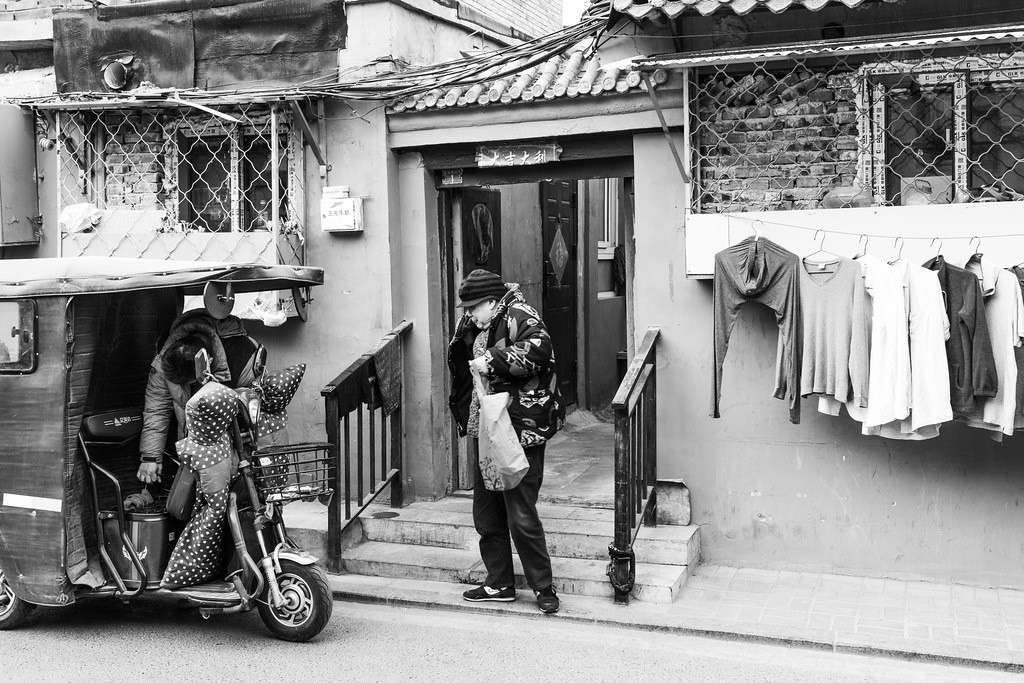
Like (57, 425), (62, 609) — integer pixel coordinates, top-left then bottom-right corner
(469, 363), (529, 492)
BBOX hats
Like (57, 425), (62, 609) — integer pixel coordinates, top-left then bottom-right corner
(455, 269), (508, 308)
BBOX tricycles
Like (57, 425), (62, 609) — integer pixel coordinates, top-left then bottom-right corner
(0, 255), (336, 642)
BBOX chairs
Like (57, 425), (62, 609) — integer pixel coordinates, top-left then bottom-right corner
(76, 404), (187, 594)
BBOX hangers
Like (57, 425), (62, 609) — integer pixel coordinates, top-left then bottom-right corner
(919, 236), (976, 281)
(714, 219), (799, 263)
(837, 234), (900, 276)
(956, 237), (1017, 280)
(885, 237), (936, 278)
(791, 229), (861, 274)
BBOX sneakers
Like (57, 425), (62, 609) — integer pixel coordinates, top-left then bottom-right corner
(534, 587), (559, 613)
(462, 584), (516, 603)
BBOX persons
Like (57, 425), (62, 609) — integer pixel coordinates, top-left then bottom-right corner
(445, 268), (565, 614)
(138, 305), (269, 485)
